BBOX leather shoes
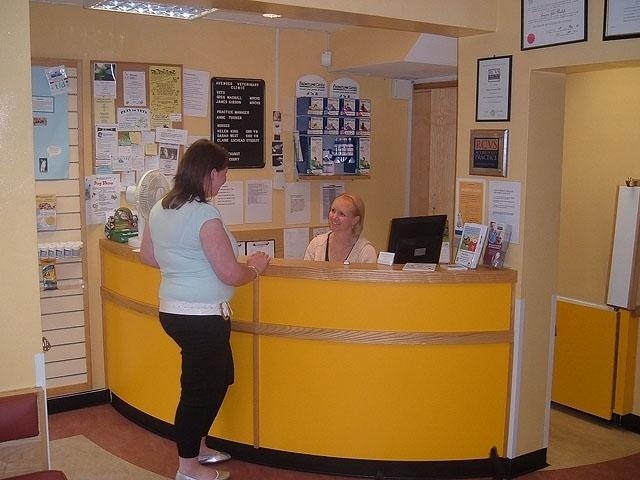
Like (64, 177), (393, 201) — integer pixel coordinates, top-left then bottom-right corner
(175, 451), (232, 480)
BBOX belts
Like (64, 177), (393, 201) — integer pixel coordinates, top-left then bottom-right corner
(159, 299), (233, 321)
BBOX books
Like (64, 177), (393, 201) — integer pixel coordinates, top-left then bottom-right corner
(440, 221), (511, 270)
(402, 261), (436, 273)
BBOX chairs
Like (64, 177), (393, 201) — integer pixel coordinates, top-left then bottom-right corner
(0, 386), (68, 480)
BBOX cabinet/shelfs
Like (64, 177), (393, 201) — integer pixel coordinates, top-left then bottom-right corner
(294, 95), (372, 181)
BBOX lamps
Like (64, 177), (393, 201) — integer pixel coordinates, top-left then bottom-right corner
(83, 0), (218, 21)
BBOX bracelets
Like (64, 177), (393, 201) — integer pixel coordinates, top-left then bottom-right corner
(247, 265), (259, 280)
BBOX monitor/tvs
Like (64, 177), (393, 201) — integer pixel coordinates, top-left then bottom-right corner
(387, 214), (447, 265)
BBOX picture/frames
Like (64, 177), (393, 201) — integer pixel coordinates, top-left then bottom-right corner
(475, 55), (513, 122)
(469, 128), (508, 177)
(602, 0), (640, 42)
(453, 177), (486, 238)
(521, 0), (588, 51)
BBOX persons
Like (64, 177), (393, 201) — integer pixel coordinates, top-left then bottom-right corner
(304, 192), (377, 263)
(139, 138), (273, 480)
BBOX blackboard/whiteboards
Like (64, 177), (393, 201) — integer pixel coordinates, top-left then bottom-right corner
(210, 76), (266, 169)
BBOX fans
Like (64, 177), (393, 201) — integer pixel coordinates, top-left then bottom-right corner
(126, 169), (170, 248)
